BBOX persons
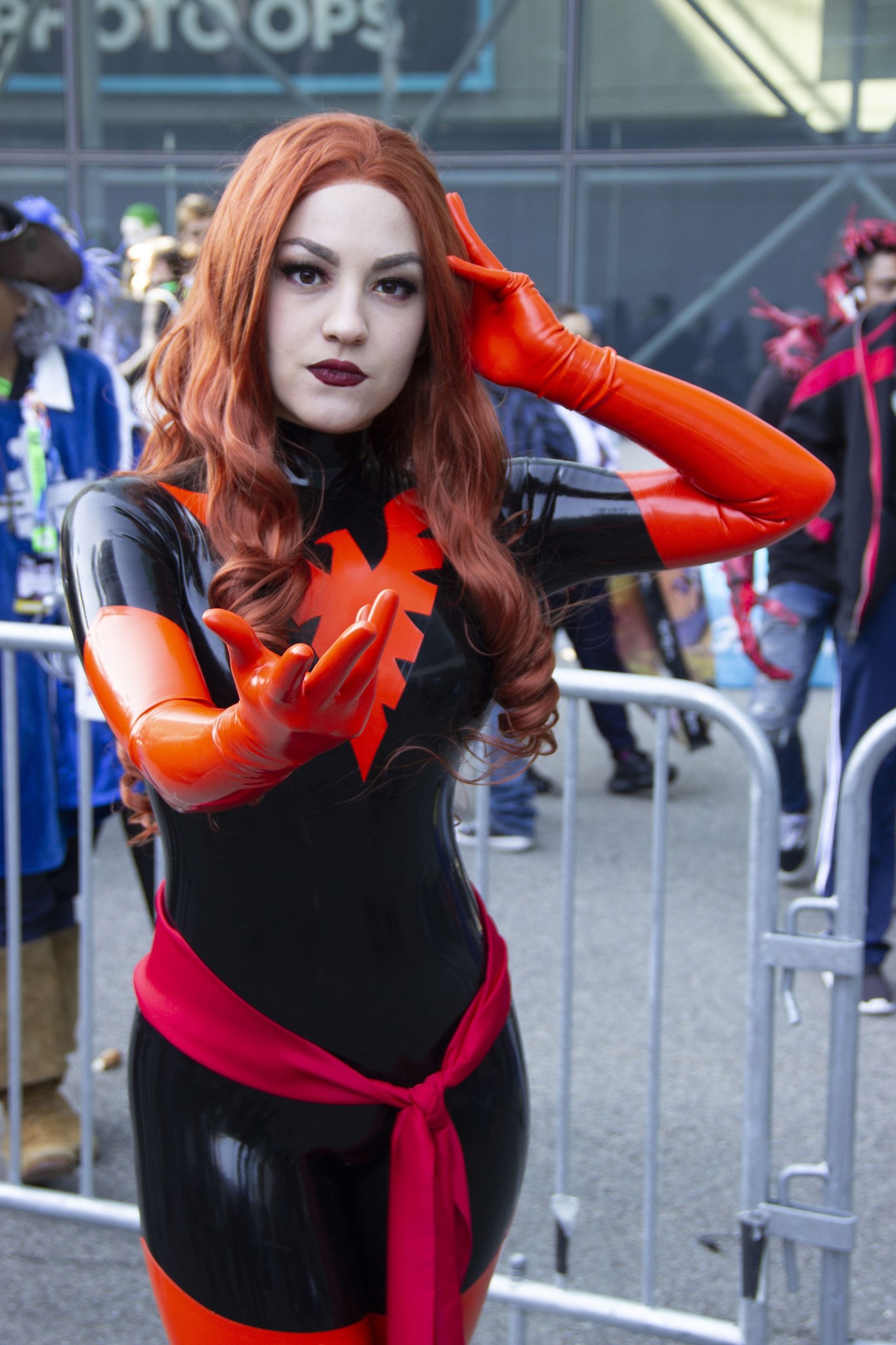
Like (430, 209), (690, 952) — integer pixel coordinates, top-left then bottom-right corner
(61, 111), (836, 1345)
(0, 189), (896, 1184)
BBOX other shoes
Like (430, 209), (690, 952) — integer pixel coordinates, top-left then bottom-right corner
(815, 927), (896, 1011)
(612, 751), (673, 797)
(772, 805), (817, 884)
(454, 811), (536, 851)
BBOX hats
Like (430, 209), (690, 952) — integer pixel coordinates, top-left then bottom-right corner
(0, 202), (82, 290)
